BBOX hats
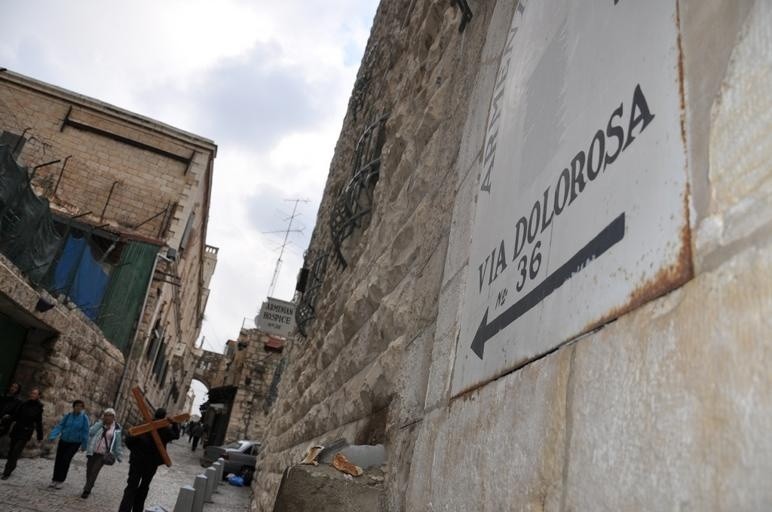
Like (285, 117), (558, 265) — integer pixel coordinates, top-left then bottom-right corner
(104, 408), (115, 414)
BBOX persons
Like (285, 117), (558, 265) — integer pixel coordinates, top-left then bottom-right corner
(118, 408), (181, 512)
(46, 399), (91, 489)
(0, 385), (45, 480)
(179, 420), (210, 451)
(81, 407), (123, 498)
(0, 382), (24, 438)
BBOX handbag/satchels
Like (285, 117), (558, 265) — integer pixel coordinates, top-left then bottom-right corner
(103, 452), (115, 465)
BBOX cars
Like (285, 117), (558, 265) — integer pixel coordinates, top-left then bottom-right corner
(197, 438), (262, 487)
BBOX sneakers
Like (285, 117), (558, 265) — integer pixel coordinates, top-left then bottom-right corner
(49, 481), (63, 489)
(81, 491), (91, 498)
